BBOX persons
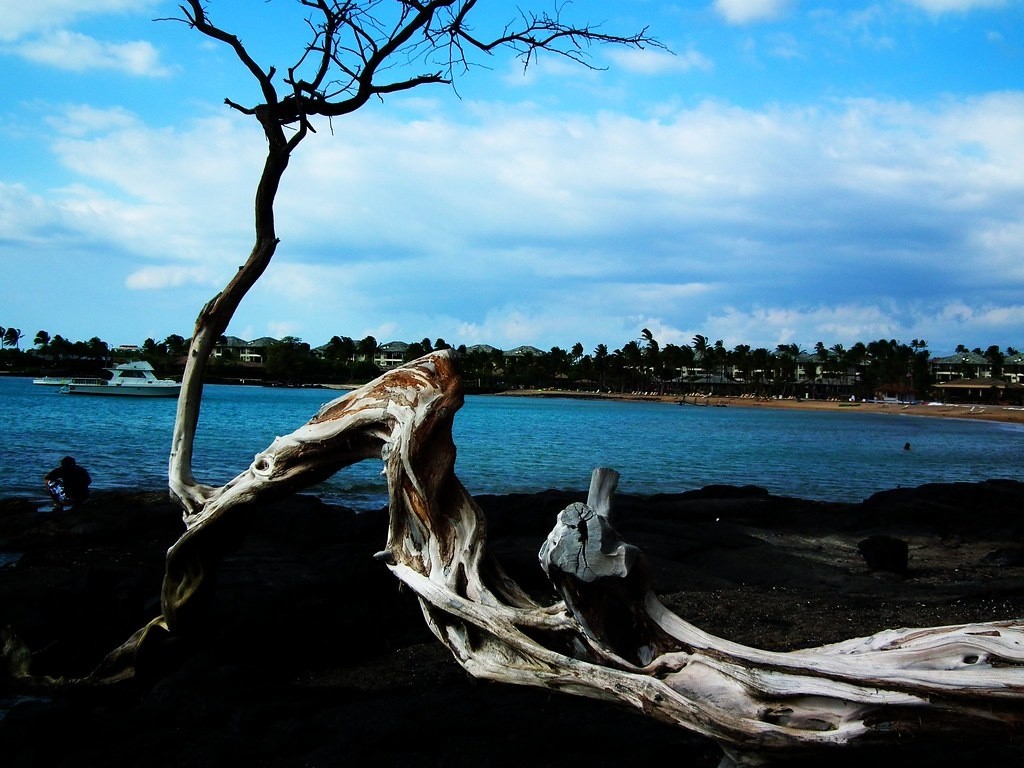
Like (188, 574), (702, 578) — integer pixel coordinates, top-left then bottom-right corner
(45, 456), (93, 510)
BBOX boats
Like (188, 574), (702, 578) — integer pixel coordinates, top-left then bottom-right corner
(64, 360), (181, 402)
(33, 377), (73, 385)
